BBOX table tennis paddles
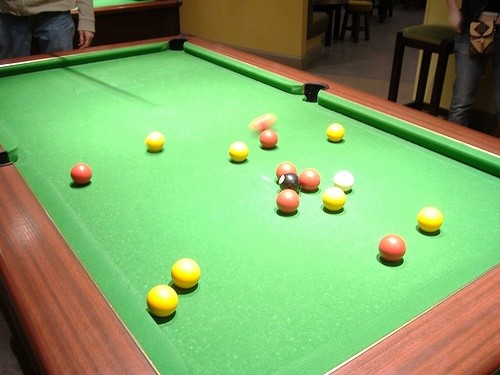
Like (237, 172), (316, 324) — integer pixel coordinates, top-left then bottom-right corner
(144, 130), (165, 152)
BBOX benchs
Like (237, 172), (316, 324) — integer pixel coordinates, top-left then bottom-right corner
(179, 0), (329, 69)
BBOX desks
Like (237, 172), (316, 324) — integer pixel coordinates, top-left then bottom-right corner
(71, 0), (180, 46)
(0, 35), (500, 375)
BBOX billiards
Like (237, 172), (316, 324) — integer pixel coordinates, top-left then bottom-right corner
(417, 205), (443, 232)
(322, 186), (346, 212)
(378, 233), (406, 262)
(333, 170), (354, 191)
(70, 162), (92, 184)
(326, 123), (345, 142)
(171, 257), (201, 289)
(275, 161), (297, 177)
(298, 168), (320, 192)
(146, 284), (178, 317)
(276, 189), (300, 213)
(229, 141), (249, 162)
(260, 129), (278, 149)
(278, 173), (300, 193)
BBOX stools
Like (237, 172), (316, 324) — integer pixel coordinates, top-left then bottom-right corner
(388, 23), (458, 118)
(338, 0), (373, 43)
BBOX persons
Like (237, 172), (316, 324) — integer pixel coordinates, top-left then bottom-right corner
(448, 0), (500, 137)
(0, 0), (95, 58)
